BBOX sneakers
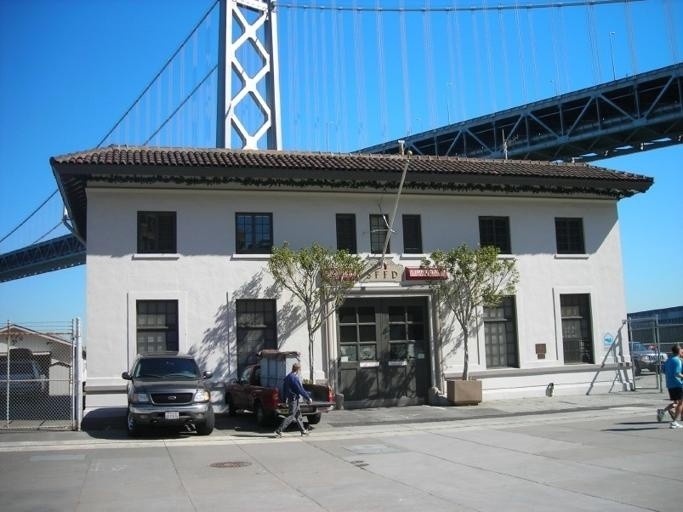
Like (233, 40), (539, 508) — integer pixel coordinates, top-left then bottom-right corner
(656, 408), (664, 422)
(669, 421), (682, 429)
(274, 430), (283, 438)
(301, 431), (311, 437)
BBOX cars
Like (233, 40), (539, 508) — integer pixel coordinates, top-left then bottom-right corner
(629, 341), (668, 376)
(0, 360), (49, 402)
(122, 350), (216, 437)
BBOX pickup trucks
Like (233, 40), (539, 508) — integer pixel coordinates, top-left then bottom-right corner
(224, 364), (336, 428)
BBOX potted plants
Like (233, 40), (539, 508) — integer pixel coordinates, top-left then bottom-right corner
(267, 239), (382, 411)
(417, 240), (521, 405)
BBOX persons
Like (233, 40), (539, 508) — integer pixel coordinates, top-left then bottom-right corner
(272, 362), (313, 438)
(666, 348), (683, 422)
(656, 346), (683, 429)
